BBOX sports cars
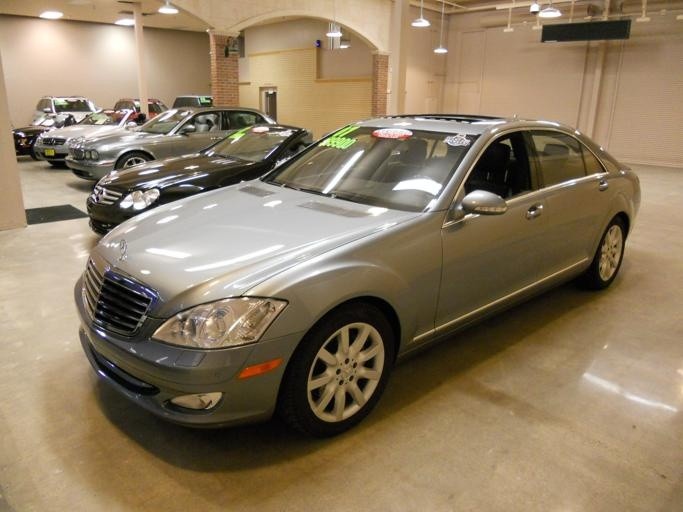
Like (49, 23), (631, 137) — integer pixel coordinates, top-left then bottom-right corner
(85, 122), (318, 232)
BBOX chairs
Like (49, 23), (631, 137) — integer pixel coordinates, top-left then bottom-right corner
(385, 138), (513, 201)
(192, 115), (209, 133)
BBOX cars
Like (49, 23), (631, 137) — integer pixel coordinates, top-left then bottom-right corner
(73, 112), (645, 436)
(63, 104), (286, 169)
(10, 86), (223, 162)
